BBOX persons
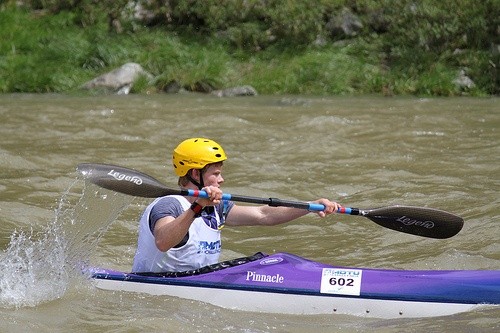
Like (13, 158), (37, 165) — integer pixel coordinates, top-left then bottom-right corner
(129, 137), (344, 276)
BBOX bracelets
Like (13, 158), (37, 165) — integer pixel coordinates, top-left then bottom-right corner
(190, 201), (204, 215)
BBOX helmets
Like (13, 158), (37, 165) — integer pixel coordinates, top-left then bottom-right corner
(173, 136), (228, 177)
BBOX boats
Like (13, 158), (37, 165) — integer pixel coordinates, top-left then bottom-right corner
(85, 251), (500, 320)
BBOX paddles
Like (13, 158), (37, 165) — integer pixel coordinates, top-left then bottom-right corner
(76, 163), (465, 240)
